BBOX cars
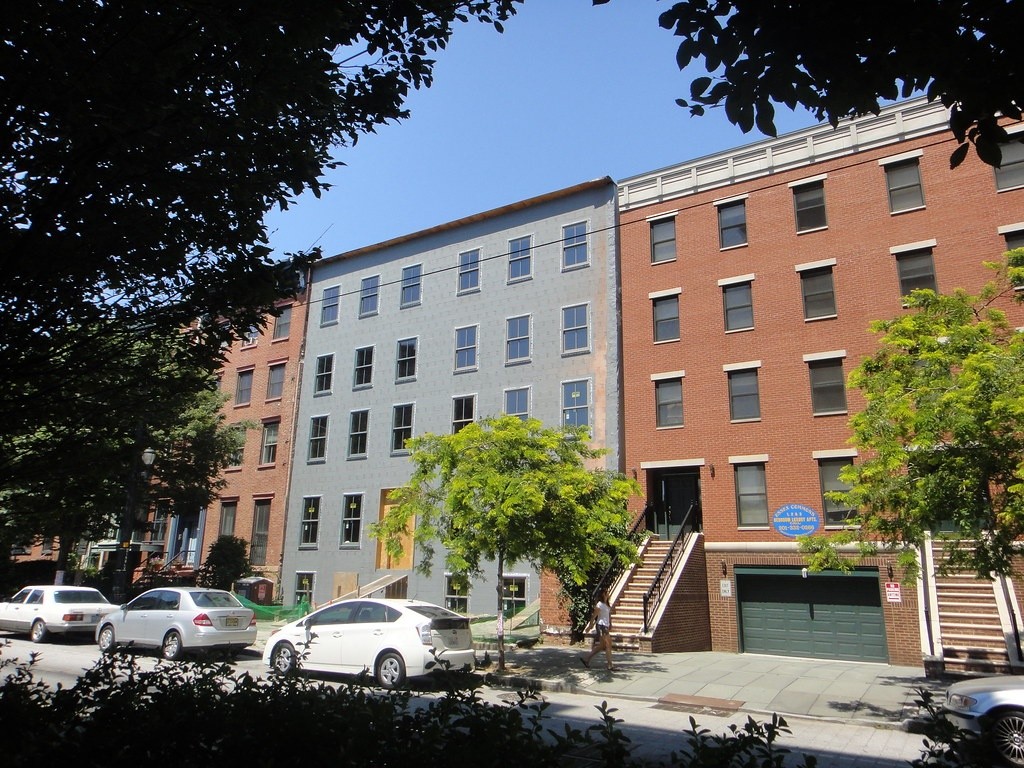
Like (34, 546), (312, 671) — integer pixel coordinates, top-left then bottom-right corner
(95, 586), (258, 664)
(0, 584), (120, 642)
(263, 598), (477, 691)
(944, 675), (1024, 768)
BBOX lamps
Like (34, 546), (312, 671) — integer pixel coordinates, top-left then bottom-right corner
(886, 561), (893, 579)
(632, 467), (637, 481)
(721, 559), (727, 577)
(708, 464), (715, 477)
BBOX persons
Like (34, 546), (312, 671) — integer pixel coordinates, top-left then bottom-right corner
(580, 587), (622, 672)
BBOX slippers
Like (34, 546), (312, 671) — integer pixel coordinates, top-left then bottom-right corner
(607, 667), (620, 671)
(580, 657), (590, 668)
